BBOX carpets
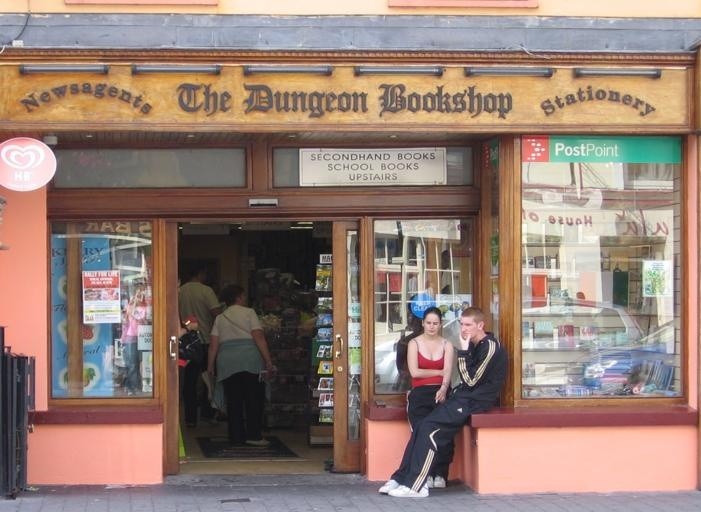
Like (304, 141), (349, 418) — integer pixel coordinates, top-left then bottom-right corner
(194, 434), (300, 462)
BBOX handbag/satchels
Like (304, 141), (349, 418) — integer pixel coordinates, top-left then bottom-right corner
(176, 330), (202, 360)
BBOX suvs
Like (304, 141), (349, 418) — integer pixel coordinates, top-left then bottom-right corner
(370, 292), (647, 394)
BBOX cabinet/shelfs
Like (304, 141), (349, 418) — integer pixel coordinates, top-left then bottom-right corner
(239, 248), (360, 450)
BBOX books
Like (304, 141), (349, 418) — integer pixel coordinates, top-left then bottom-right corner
(522, 321), (680, 397)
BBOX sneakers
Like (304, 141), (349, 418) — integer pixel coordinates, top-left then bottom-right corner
(245, 437), (273, 446)
(433, 475), (446, 489)
(187, 421), (199, 428)
(425, 475), (434, 489)
(377, 478), (400, 493)
(388, 482), (430, 498)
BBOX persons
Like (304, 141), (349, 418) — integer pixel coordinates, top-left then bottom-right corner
(177, 259), (222, 429)
(120, 281), (147, 396)
(405, 306), (456, 488)
(441, 248), (453, 294)
(376, 306), (508, 497)
(206, 283), (273, 448)
(176, 277), (198, 458)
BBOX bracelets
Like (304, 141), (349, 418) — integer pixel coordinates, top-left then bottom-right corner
(183, 326), (188, 334)
(442, 381), (448, 389)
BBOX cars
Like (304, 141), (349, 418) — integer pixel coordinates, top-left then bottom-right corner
(562, 317), (674, 397)
